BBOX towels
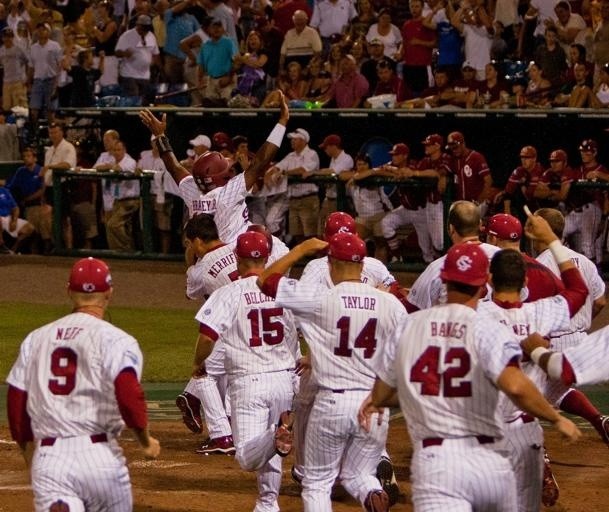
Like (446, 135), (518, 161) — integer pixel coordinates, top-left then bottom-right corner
(153, 170), (166, 206)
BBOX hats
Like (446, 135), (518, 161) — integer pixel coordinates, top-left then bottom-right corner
(389, 130), (464, 154)
(321, 211), (359, 242)
(439, 242), (489, 286)
(325, 232), (367, 264)
(234, 231), (270, 258)
(290, 127), (342, 149)
(519, 139), (597, 163)
(69, 256), (112, 293)
(151, 129), (228, 149)
(479, 213), (523, 241)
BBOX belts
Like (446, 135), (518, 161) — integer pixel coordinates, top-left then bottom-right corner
(422, 435), (495, 447)
(40, 431), (108, 446)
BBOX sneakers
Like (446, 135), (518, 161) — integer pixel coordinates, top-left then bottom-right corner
(376, 457), (400, 507)
(292, 463), (305, 484)
(594, 413), (609, 446)
(273, 410), (296, 457)
(540, 454), (559, 507)
(48, 498), (71, 512)
(195, 435), (237, 455)
(175, 391), (204, 435)
(364, 489), (390, 512)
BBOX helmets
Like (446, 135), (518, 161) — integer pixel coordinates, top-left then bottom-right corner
(192, 151), (234, 194)
